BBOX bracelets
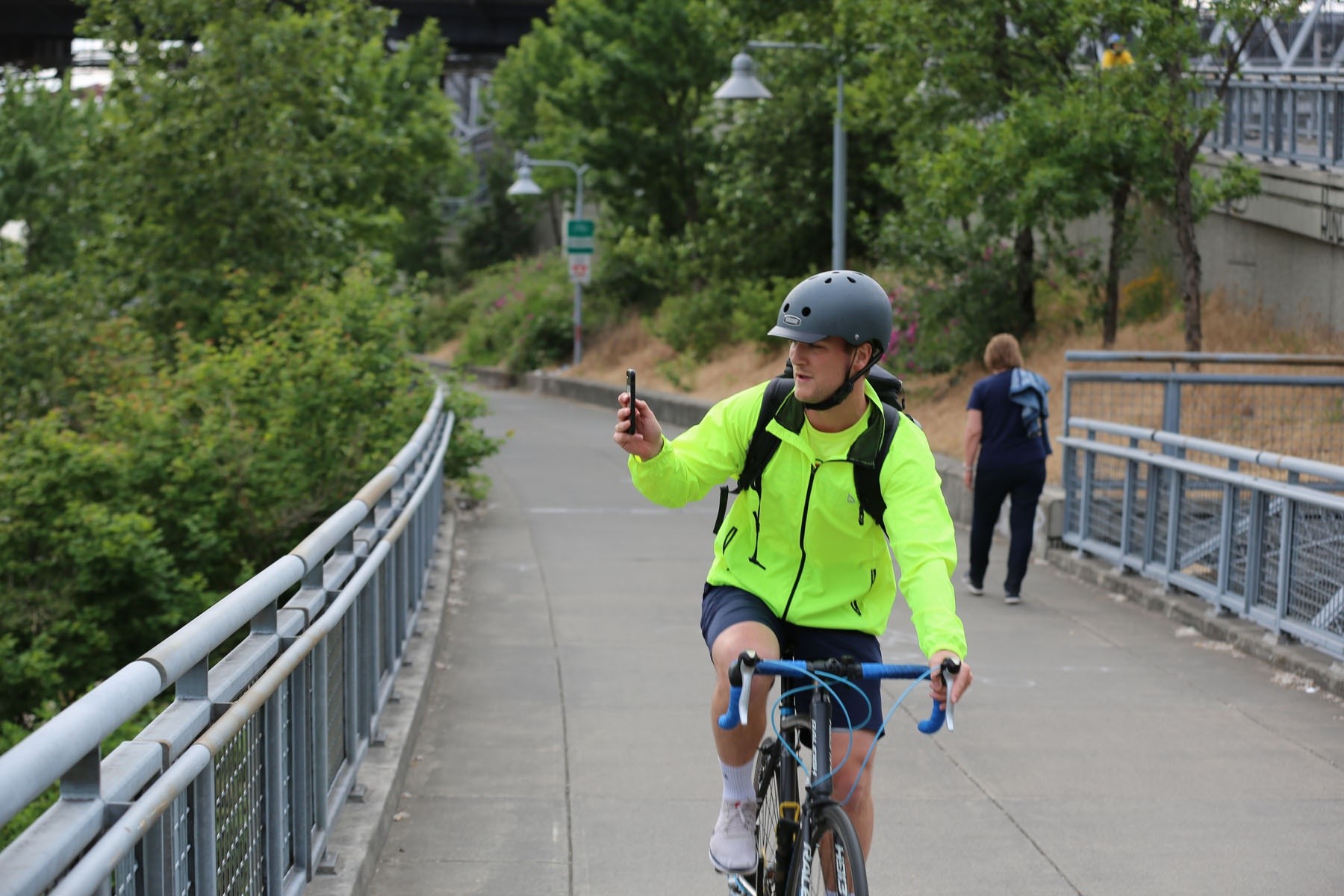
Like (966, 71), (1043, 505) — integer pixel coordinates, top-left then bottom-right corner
(961, 461), (974, 473)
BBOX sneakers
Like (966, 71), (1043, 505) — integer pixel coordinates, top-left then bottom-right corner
(708, 797), (760, 875)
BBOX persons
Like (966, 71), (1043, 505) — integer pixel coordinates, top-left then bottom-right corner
(609, 268), (973, 893)
(959, 332), (1052, 605)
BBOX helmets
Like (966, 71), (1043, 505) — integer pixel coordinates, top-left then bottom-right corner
(766, 270), (893, 355)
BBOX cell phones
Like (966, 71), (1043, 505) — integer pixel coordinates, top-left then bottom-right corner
(626, 369), (635, 435)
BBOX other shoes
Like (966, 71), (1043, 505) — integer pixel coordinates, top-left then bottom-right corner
(961, 571), (983, 595)
(1004, 589), (1021, 604)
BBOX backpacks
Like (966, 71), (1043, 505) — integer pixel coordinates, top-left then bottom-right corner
(712, 358), (905, 541)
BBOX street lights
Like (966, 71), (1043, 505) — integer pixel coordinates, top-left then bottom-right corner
(710, 39), (848, 270)
(506, 147), (596, 370)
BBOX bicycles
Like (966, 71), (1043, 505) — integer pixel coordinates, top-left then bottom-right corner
(714, 651), (964, 894)
